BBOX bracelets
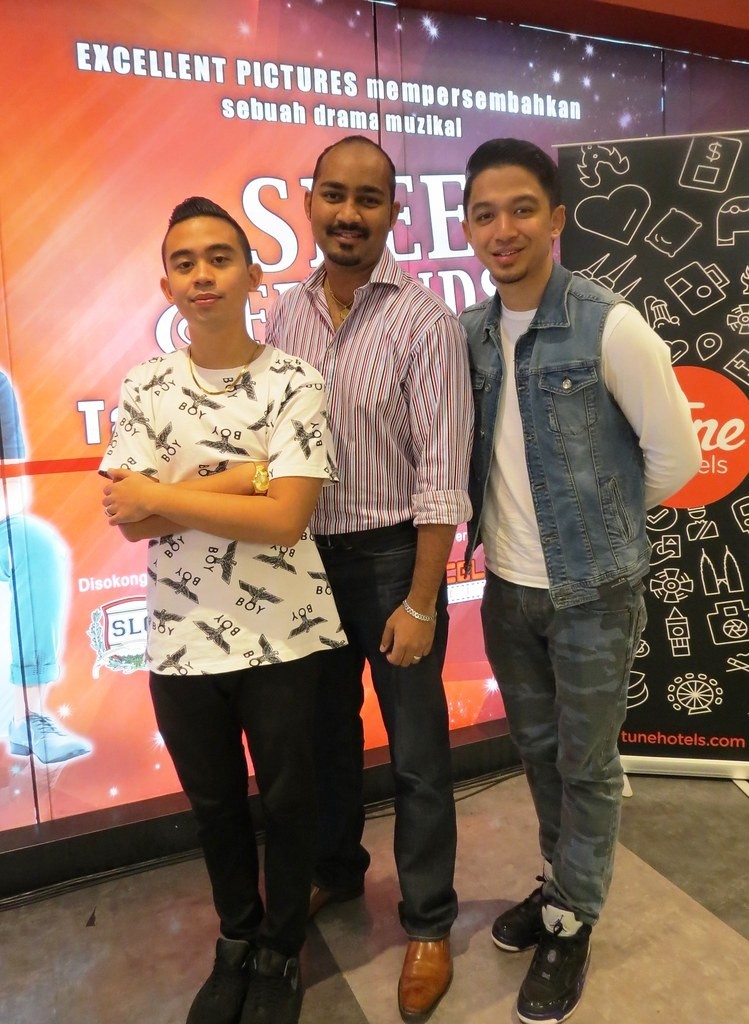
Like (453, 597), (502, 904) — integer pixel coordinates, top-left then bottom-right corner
(402, 598), (438, 622)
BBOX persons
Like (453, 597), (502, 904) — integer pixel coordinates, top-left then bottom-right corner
(0, 371), (93, 764)
(457, 138), (703, 1024)
(264, 136), (475, 1024)
(99, 197), (350, 1024)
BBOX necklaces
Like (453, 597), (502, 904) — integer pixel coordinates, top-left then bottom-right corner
(325, 276), (356, 320)
(187, 339), (261, 395)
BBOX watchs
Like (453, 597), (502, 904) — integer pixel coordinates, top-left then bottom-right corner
(252, 461), (270, 496)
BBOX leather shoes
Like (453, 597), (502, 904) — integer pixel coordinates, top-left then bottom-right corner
(307, 884), (335, 915)
(8, 714), (92, 765)
(398, 940), (453, 1020)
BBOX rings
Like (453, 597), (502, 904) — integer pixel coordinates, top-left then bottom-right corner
(413, 654), (422, 661)
(104, 507), (112, 517)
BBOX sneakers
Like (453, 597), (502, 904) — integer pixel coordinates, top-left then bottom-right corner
(491, 876), (553, 952)
(516, 900), (592, 1023)
(186, 936), (246, 1023)
(245, 930), (304, 1024)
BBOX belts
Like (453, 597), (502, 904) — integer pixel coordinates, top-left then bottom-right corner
(311, 518), (418, 550)
